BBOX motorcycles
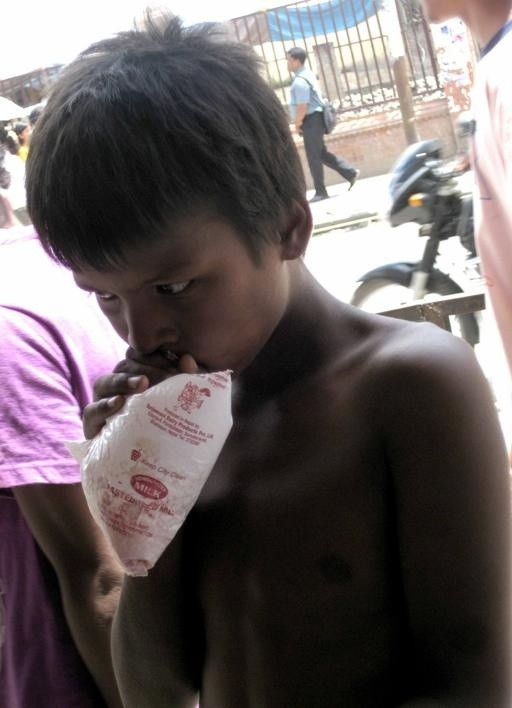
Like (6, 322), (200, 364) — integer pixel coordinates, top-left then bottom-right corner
(349, 115), (505, 352)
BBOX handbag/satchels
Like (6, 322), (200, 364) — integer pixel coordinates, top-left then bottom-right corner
(323, 105), (336, 134)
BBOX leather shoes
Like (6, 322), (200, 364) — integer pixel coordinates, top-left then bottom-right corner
(309, 195), (329, 202)
(348, 172), (358, 191)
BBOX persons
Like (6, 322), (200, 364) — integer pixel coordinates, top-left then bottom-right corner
(0, 197), (132, 706)
(0, 105), (45, 226)
(419, 0), (512, 374)
(25, 4), (512, 705)
(282, 44), (360, 204)
